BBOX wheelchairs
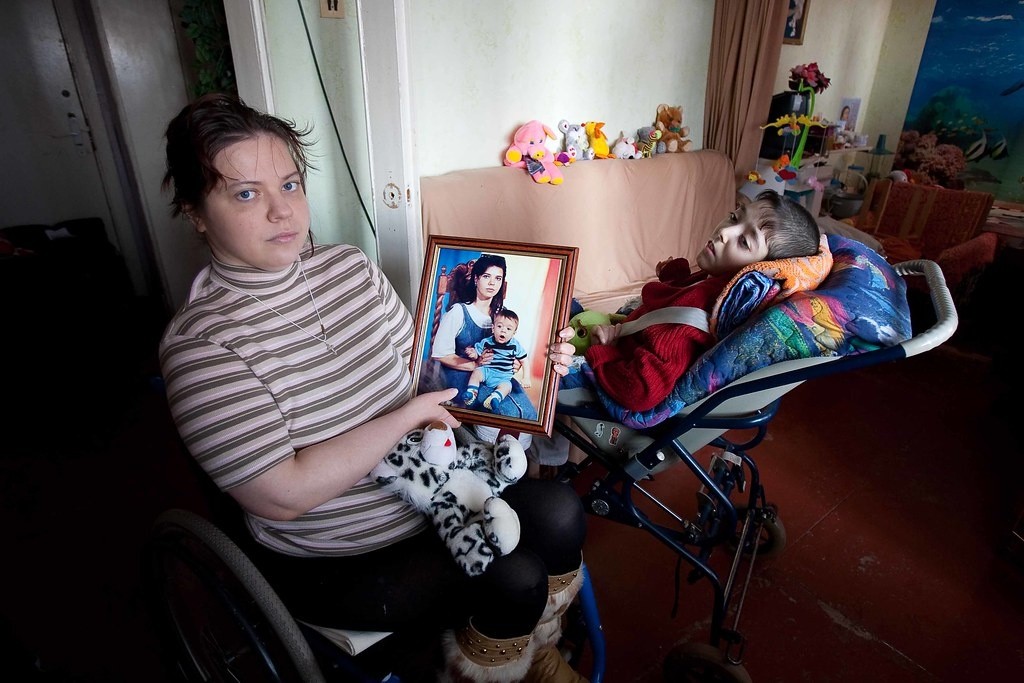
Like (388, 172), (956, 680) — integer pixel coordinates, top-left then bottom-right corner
(140, 409), (607, 682)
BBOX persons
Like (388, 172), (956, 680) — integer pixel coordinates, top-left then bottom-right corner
(431, 253), (537, 421)
(475, 189), (820, 465)
(784, 0), (805, 36)
(159, 94), (586, 683)
(840, 106), (851, 131)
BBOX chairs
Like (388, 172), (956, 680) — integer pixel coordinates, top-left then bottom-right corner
(564, 238), (912, 475)
(878, 179), (996, 263)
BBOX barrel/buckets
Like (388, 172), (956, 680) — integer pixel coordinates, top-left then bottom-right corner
(832, 195), (864, 220)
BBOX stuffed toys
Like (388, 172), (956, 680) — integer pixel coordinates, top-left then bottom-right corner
(369, 422), (527, 576)
(887, 169), (915, 183)
(568, 308), (628, 356)
(504, 104), (693, 185)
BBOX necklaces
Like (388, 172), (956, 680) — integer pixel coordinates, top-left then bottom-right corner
(212, 260), (337, 356)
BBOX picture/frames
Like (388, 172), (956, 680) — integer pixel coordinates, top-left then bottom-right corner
(408, 234), (580, 438)
(782, 0), (811, 45)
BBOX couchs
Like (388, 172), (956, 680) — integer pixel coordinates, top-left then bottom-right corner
(419, 150), (736, 314)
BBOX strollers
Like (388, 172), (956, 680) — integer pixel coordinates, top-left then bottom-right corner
(552, 258), (960, 683)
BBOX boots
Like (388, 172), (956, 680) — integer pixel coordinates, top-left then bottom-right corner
(528, 550), (591, 683)
(439, 615), (534, 683)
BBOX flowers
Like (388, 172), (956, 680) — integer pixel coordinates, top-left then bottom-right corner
(788, 62), (831, 94)
